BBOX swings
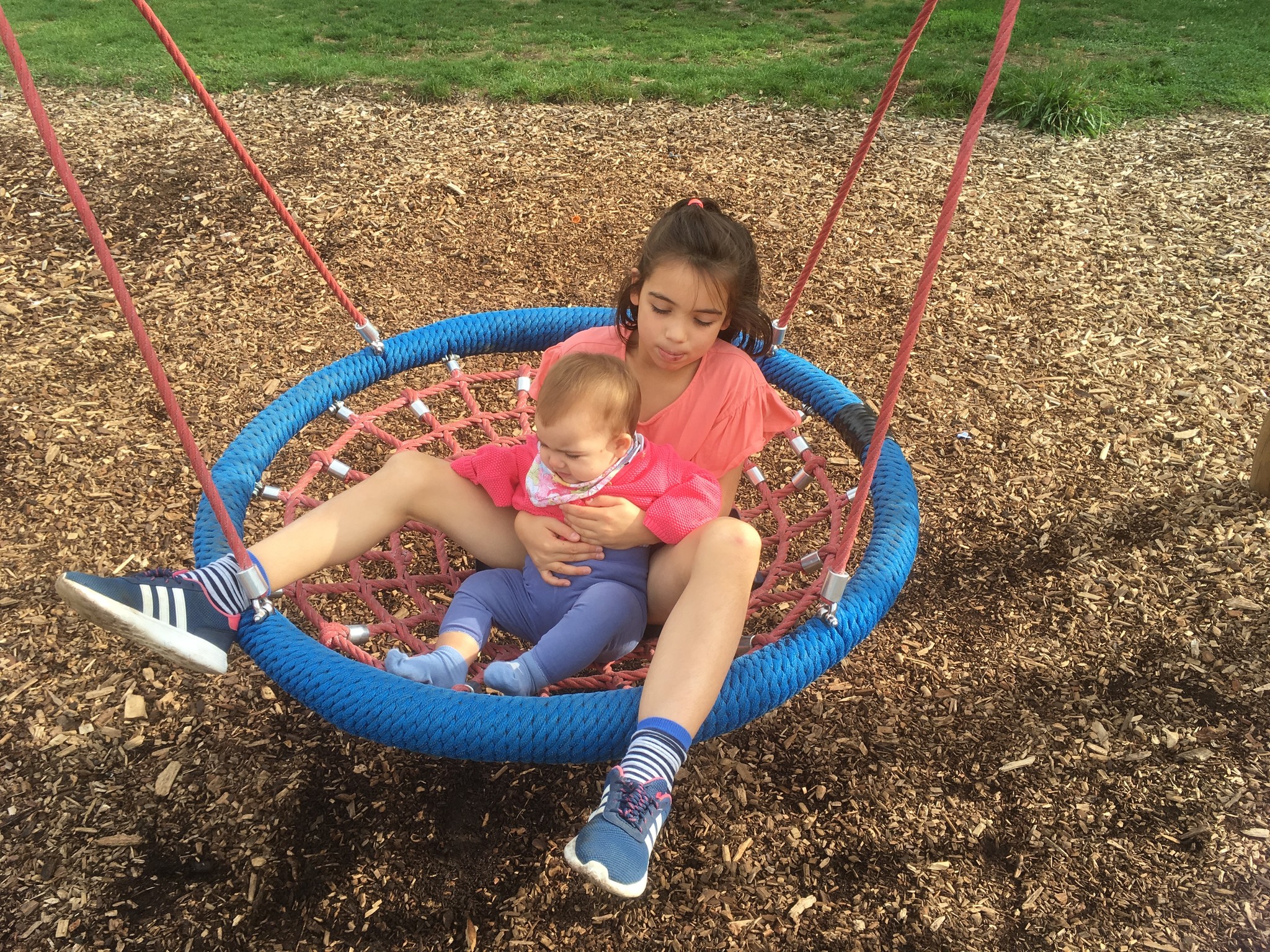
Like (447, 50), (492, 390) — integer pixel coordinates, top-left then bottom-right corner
(0, 0), (1024, 767)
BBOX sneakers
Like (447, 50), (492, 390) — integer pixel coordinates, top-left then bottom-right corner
(562, 764), (672, 897)
(54, 567), (238, 675)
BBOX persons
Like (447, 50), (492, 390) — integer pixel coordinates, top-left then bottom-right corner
(51, 197), (804, 896)
(385, 351), (720, 696)
